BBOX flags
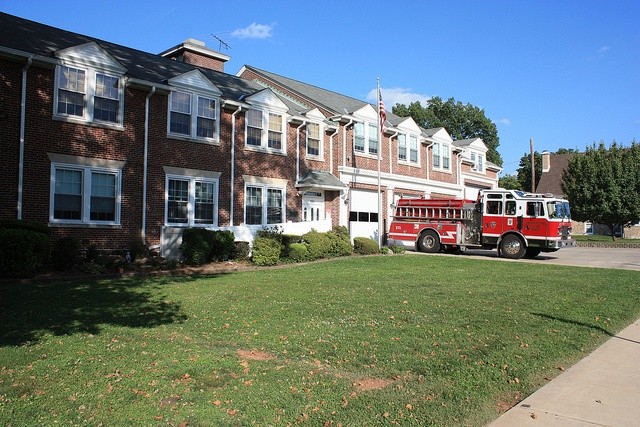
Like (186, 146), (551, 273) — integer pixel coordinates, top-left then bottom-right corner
(379, 89), (387, 131)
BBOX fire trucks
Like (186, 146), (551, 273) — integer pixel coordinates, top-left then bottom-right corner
(387, 189), (575, 258)
